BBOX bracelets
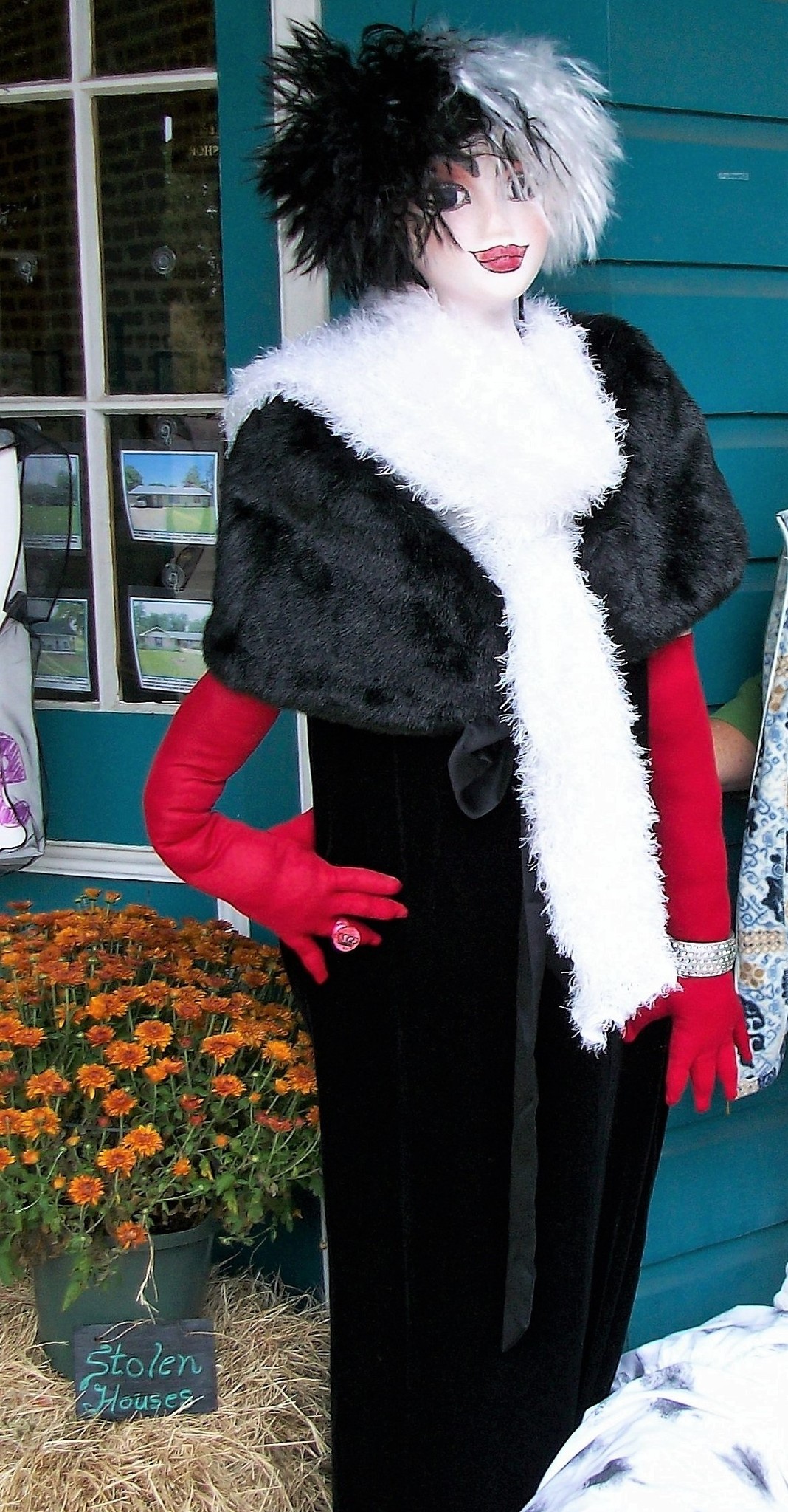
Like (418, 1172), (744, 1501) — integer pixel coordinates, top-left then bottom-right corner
(668, 930), (735, 978)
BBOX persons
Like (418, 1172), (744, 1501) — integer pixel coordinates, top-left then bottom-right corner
(142, 5), (762, 1512)
(706, 676), (760, 793)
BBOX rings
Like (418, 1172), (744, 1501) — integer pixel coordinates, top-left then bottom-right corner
(332, 916), (360, 952)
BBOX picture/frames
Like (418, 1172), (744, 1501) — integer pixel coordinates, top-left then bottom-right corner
(16, 436), (224, 698)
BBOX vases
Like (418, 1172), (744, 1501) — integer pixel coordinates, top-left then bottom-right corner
(37, 1187), (223, 1383)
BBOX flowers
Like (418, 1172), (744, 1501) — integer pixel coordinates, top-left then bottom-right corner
(2, 885), (329, 1314)
